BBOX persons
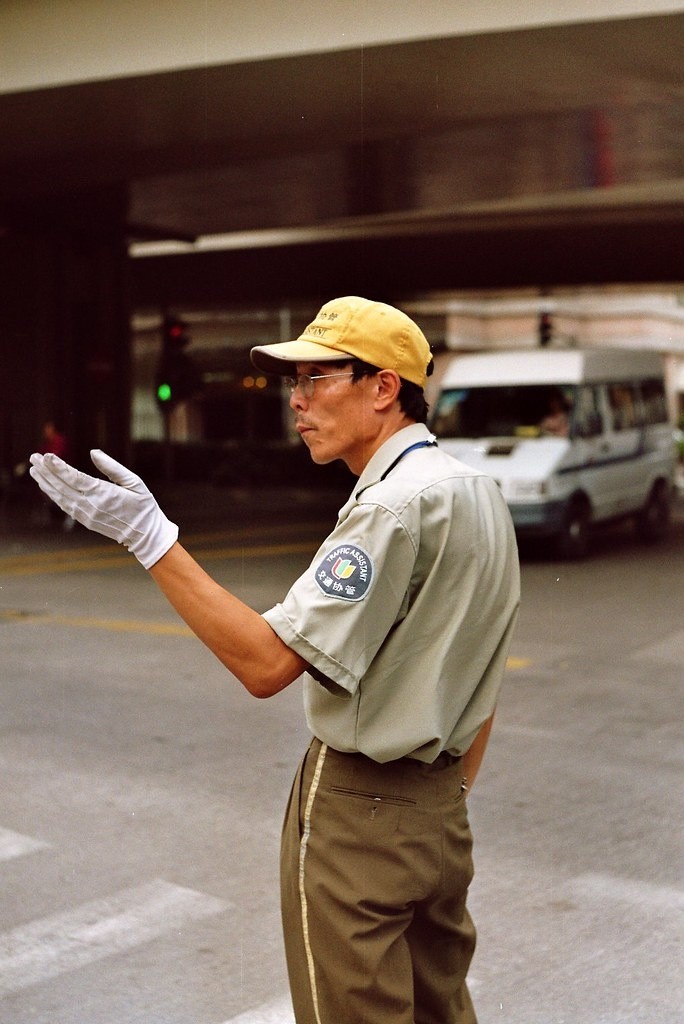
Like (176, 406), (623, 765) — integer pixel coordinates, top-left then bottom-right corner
(38, 422), (63, 457)
(539, 403), (575, 440)
(27, 295), (522, 1024)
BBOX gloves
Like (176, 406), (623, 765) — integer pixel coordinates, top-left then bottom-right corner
(29, 449), (179, 571)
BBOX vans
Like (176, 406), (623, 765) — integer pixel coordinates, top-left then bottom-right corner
(426, 337), (676, 562)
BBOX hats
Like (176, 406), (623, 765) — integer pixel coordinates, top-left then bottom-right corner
(249, 296), (433, 393)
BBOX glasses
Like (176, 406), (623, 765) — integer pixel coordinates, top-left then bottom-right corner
(284, 372), (354, 398)
